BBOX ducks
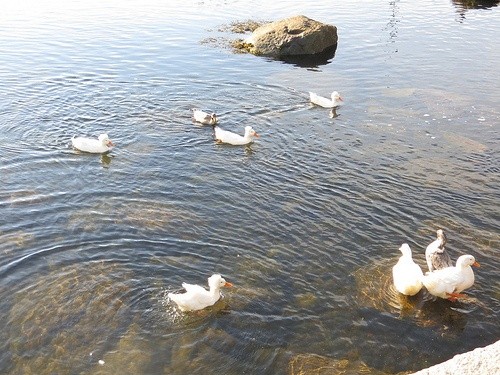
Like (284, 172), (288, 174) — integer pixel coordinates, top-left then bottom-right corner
(421, 255), (480, 301)
(193, 110), (218, 125)
(308, 91), (343, 108)
(71, 134), (113, 153)
(426, 230), (453, 272)
(168, 274), (235, 312)
(214, 125), (260, 146)
(393, 243), (424, 296)
